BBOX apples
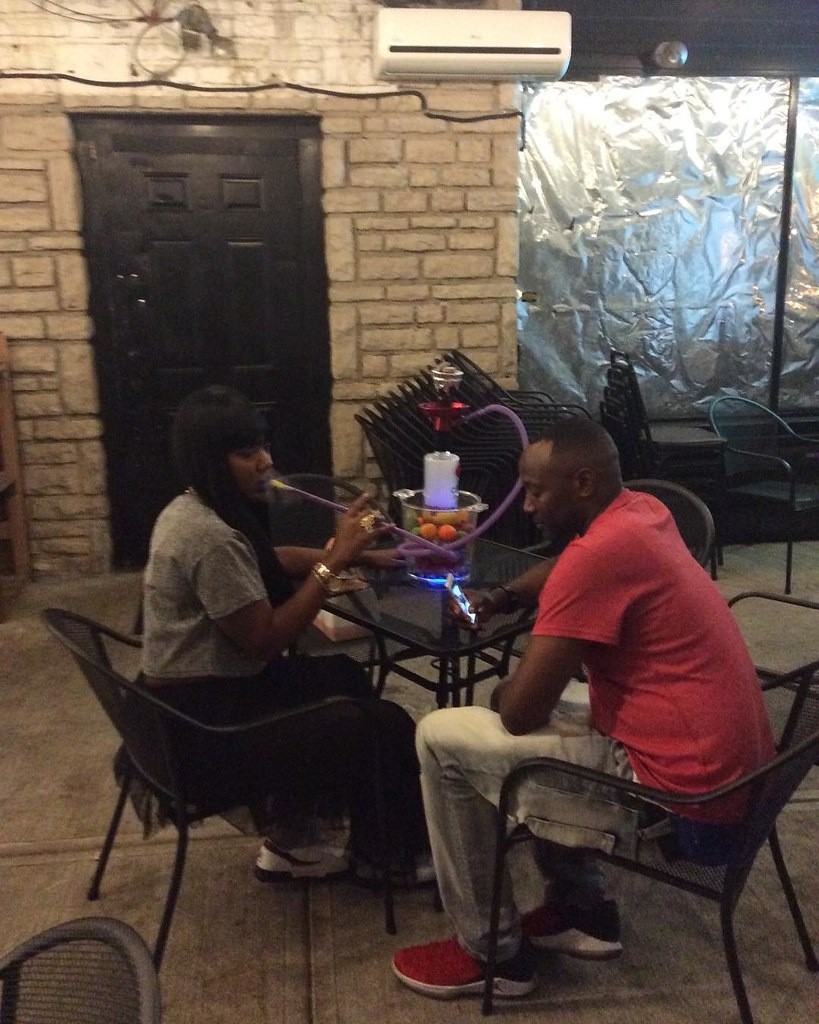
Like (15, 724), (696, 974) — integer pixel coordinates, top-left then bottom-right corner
(417, 523), (458, 542)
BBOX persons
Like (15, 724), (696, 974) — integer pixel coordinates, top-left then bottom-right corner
(139, 387), (440, 887)
(391, 418), (776, 999)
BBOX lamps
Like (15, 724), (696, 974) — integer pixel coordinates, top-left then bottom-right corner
(644, 39), (688, 71)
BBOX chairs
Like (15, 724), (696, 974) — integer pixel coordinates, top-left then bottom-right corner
(711, 395), (819, 595)
(265, 471), (412, 684)
(0, 915), (159, 1024)
(354, 348), (593, 529)
(600, 350), (730, 580)
(38, 607), (396, 972)
(481, 592), (819, 1024)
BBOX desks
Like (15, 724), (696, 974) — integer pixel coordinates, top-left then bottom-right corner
(293, 534), (549, 709)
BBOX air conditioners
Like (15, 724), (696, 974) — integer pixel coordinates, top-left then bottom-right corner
(372, 9), (572, 83)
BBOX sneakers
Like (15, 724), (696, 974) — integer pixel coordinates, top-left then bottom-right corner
(347, 849), (436, 888)
(253, 832), (347, 883)
(391, 936), (539, 997)
(520, 900), (623, 960)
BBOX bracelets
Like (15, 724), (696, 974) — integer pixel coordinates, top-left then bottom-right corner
(500, 583), (519, 612)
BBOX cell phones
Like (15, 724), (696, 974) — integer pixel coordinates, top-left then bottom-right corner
(445, 572), (480, 629)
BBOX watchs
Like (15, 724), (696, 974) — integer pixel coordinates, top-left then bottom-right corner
(312, 559), (345, 595)
